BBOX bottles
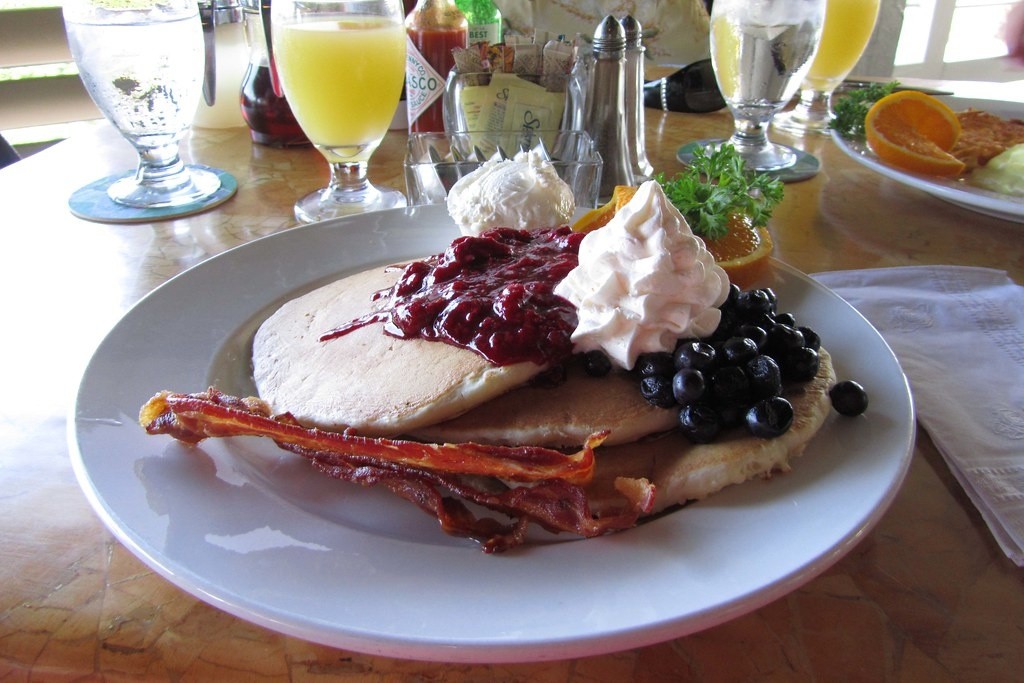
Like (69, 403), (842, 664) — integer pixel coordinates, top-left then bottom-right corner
(407, 0), (503, 133)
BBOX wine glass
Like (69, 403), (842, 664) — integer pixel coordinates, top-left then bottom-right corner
(773, 1), (880, 132)
(273, 1), (412, 219)
(50, 1), (238, 222)
(676, 0), (825, 170)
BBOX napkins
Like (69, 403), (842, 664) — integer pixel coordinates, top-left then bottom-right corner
(805, 262), (1023, 567)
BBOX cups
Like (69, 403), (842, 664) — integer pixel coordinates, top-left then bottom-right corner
(199, 0), (311, 148)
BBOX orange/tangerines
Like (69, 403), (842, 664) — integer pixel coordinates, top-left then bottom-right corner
(864, 90), (965, 178)
(569, 185), (773, 291)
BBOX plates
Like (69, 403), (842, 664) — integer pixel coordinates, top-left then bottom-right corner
(69, 165), (238, 225)
(830, 92), (1024, 225)
(675, 140), (822, 182)
(72, 199), (917, 664)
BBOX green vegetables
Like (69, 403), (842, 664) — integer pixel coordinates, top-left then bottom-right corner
(826, 78), (905, 141)
(659, 140), (787, 245)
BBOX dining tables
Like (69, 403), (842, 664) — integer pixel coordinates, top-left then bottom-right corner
(0, 65), (1023, 683)
